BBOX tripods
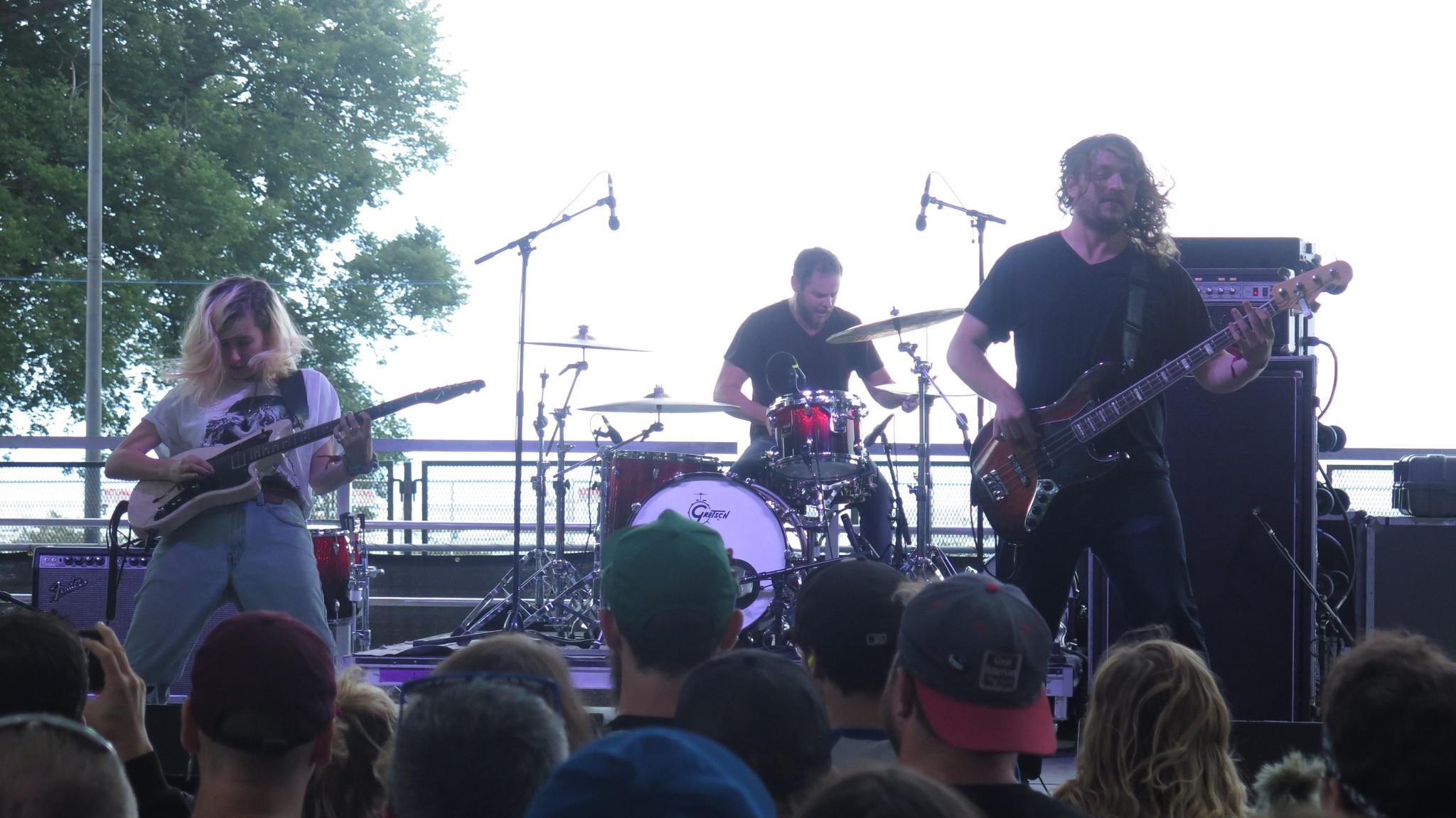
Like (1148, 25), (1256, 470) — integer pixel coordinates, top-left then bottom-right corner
(447, 337), (961, 645)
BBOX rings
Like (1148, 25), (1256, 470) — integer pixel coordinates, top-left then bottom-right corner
(336, 435), (344, 443)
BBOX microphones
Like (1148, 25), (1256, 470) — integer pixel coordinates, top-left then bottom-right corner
(957, 412), (972, 456)
(608, 175), (619, 231)
(915, 174), (930, 232)
(602, 416), (623, 444)
(790, 355), (808, 386)
(863, 414), (895, 446)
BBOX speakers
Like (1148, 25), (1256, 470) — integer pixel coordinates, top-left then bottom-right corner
(32, 546), (243, 704)
(1080, 354), (1320, 722)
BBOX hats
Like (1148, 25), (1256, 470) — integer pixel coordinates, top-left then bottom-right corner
(601, 508), (738, 635)
(786, 558), (903, 666)
(185, 613), (342, 748)
(896, 569), (1058, 755)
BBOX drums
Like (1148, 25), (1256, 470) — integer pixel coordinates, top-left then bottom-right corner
(766, 390), (876, 483)
(623, 471), (809, 633)
(791, 477), (867, 505)
(303, 519), (367, 626)
(597, 445), (722, 539)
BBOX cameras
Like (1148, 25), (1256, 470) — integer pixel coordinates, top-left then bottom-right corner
(75, 626), (112, 690)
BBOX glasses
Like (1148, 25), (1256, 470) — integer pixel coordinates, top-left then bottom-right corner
(396, 672), (559, 744)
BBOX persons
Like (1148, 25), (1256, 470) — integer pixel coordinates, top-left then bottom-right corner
(105, 275), (373, 706)
(946, 132), (1276, 784)
(0, 507), (1456, 818)
(712, 248), (896, 564)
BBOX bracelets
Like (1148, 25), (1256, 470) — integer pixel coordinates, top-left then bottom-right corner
(342, 452), (380, 475)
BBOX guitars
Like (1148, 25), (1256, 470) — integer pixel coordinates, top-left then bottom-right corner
(126, 380), (485, 537)
(970, 261), (1354, 545)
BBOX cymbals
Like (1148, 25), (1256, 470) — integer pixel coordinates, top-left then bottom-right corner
(825, 306), (966, 343)
(874, 383), (977, 396)
(576, 385), (742, 412)
(520, 325), (648, 352)
